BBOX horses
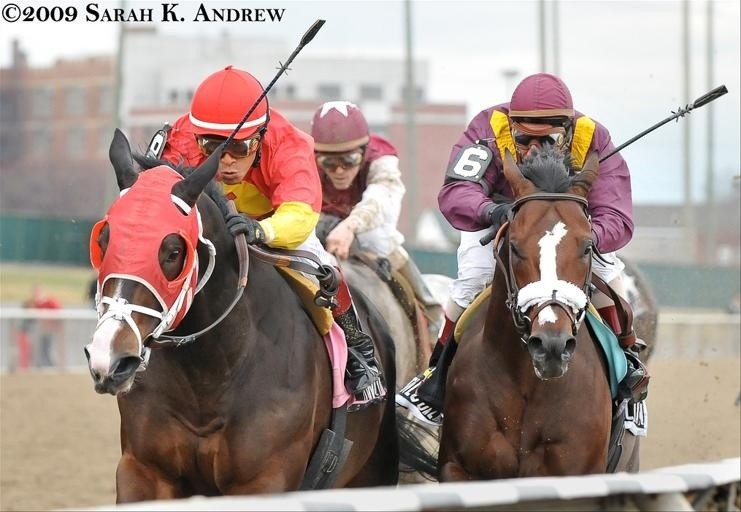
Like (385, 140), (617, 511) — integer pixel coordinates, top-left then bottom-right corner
(435, 148), (658, 511)
(82, 127), (449, 504)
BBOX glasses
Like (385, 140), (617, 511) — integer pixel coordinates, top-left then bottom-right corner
(314, 151), (363, 172)
(512, 129), (568, 153)
(195, 135), (260, 159)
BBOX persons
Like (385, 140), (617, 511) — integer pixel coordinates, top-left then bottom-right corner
(21, 283), (63, 368)
(310, 101), (445, 352)
(416, 71), (650, 409)
(144, 65), (392, 413)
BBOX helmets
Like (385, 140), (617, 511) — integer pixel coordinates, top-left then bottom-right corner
(189, 68), (269, 140)
(311, 100), (370, 154)
(507, 71), (576, 127)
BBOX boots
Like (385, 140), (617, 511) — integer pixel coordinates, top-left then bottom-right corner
(336, 300), (383, 392)
(398, 255), (442, 310)
